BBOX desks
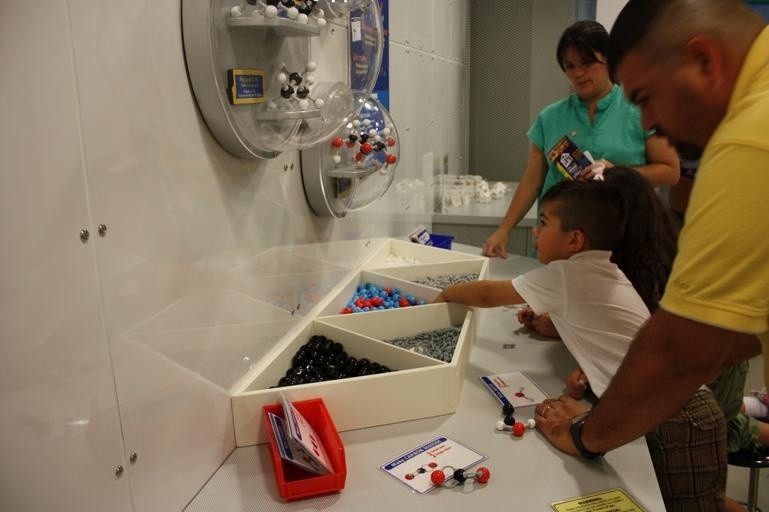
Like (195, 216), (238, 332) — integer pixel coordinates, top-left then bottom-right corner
(434, 178), (542, 255)
(182, 239), (671, 511)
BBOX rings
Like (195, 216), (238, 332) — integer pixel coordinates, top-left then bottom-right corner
(541, 405), (550, 417)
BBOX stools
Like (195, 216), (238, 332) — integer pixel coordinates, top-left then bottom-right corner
(729, 449), (768, 511)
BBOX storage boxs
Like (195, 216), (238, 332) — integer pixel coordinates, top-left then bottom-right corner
(229, 238), (489, 450)
(262, 398), (348, 503)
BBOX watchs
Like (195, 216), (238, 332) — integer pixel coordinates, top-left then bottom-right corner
(570, 411), (605, 458)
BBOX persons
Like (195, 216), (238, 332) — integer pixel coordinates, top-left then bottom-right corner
(533, 0), (769, 459)
(592, 167), (768, 458)
(431, 180), (748, 511)
(481, 19), (681, 261)
